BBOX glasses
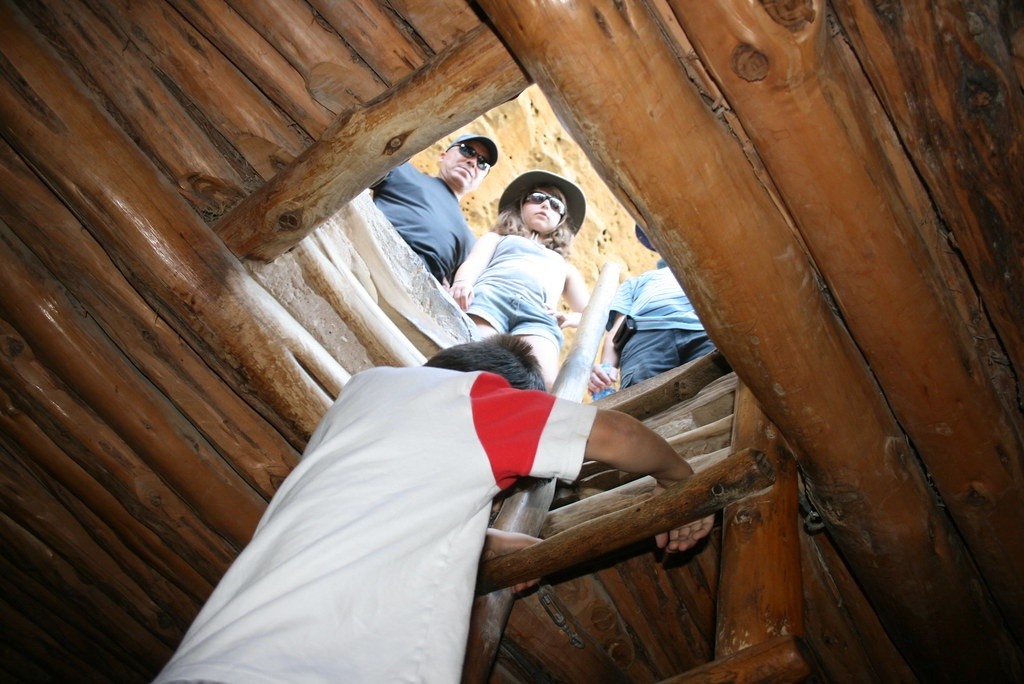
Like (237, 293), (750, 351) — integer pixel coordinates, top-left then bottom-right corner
(453, 143), (490, 170)
(523, 190), (565, 216)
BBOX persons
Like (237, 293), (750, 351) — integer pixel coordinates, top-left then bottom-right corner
(588, 223), (715, 396)
(450, 170), (590, 391)
(152, 333), (715, 684)
(368, 134), (498, 292)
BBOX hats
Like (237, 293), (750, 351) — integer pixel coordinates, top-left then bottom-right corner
(498, 169), (587, 247)
(445, 134), (499, 168)
